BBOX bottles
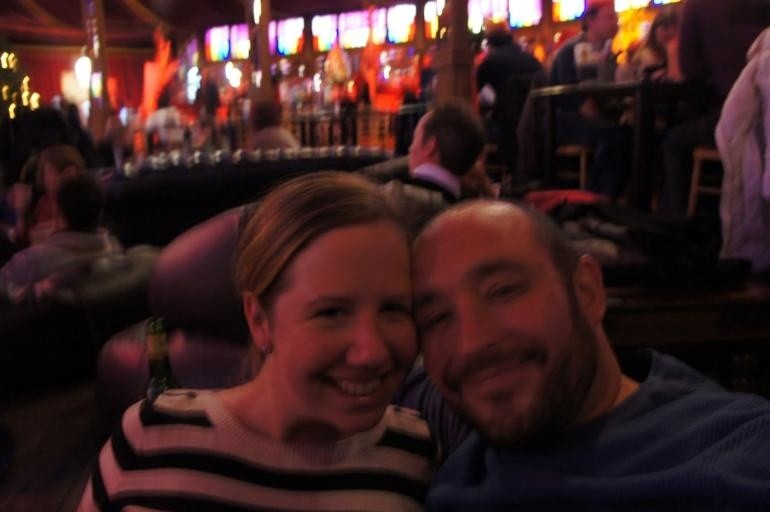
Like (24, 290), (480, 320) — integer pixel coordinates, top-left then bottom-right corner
(143, 316), (182, 402)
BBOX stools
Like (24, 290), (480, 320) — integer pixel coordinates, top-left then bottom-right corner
(679, 148), (724, 216)
(554, 144), (591, 189)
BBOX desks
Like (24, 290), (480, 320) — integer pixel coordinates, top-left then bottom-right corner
(527, 76), (696, 210)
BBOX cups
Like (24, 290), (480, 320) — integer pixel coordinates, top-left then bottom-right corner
(123, 145), (382, 180)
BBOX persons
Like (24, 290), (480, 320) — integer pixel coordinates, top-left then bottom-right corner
(389, 199), (768, 512)
(78, 172), (441, 511)
(1, 3), (768, 300)
